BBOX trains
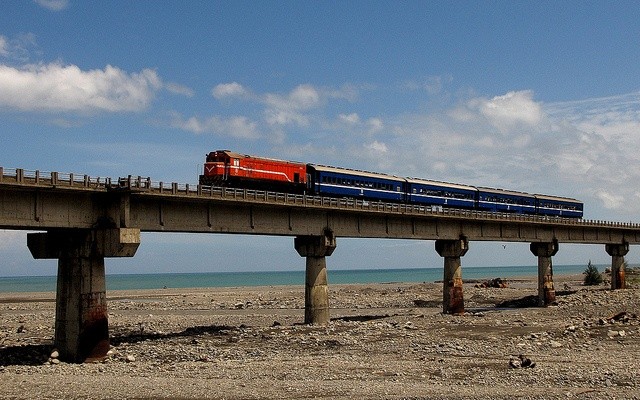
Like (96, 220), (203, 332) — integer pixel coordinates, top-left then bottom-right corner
(199, 149), (583, 220)
